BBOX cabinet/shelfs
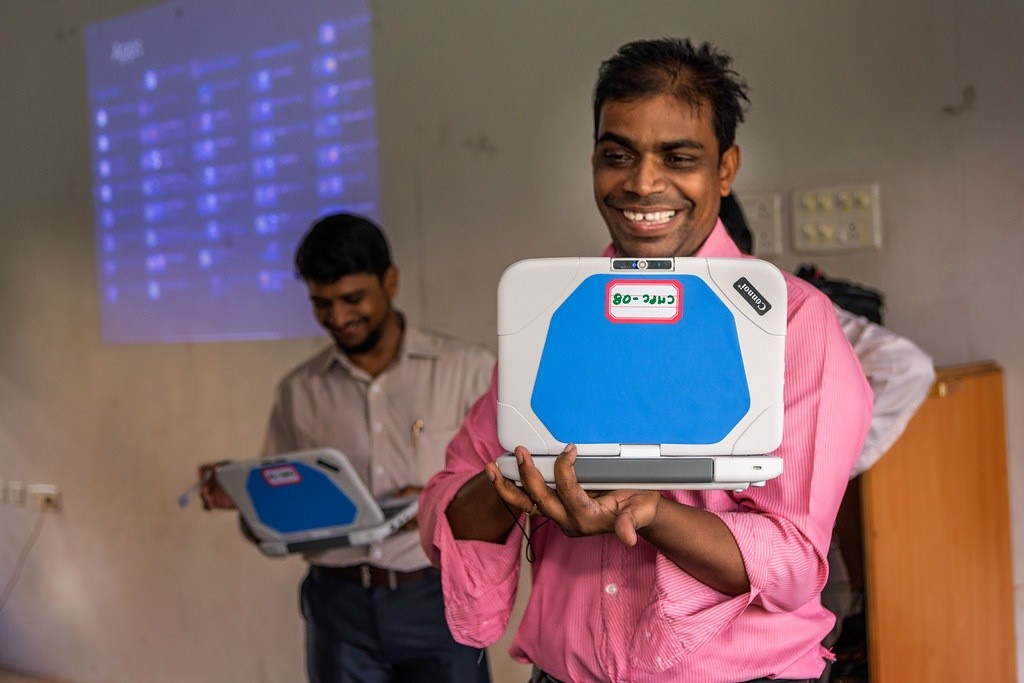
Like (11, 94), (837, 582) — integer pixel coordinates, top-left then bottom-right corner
(860, 359), (1021, 683)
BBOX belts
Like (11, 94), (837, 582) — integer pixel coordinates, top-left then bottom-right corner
(309, 564), (444, 592)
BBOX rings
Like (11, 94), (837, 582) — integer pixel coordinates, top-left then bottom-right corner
(526, 502), (536, 515)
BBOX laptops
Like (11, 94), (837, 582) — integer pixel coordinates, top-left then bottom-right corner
(214, 447), (421, 559)
(496, 256), (787, 490)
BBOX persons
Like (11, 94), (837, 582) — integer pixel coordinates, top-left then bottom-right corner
(418, 41), (877, 683)
(718, 186), (935, 683)
(197, 213), (498, 683)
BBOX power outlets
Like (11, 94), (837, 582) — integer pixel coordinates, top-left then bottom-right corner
(24, 484), (63, 512)
